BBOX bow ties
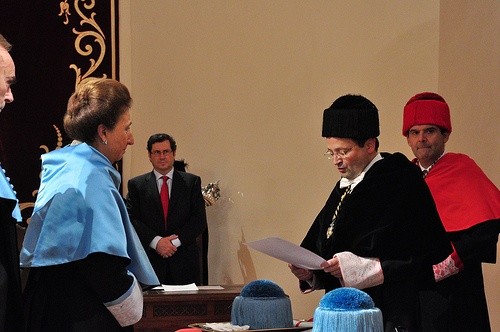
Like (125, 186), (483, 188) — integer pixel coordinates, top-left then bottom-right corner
(340, 172), (365, 188)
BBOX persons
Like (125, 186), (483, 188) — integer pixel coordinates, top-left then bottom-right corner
(126, 133), (207, 285)
(0, 33), (26, 332)
(402, 91), (500, 332)
(20, 76), (161, 332)
(287, 94), (455, 332)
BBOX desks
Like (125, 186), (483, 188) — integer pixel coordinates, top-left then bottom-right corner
(133, 283), (244, 332)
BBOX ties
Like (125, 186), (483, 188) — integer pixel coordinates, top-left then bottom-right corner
(159, 176), (170, 227)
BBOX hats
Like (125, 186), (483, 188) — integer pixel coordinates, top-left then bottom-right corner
(402, 92), (453, 137)
(322, 93), (380, 139)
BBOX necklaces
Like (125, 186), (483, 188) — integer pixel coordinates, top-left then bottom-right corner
(327, 186), (352, 238)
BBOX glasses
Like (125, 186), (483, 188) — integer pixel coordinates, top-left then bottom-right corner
(324, 144), (357, 160)
(151, 150), (172, 156)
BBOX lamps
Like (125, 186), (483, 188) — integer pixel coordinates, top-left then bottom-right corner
(202, 180), (222, 207)
